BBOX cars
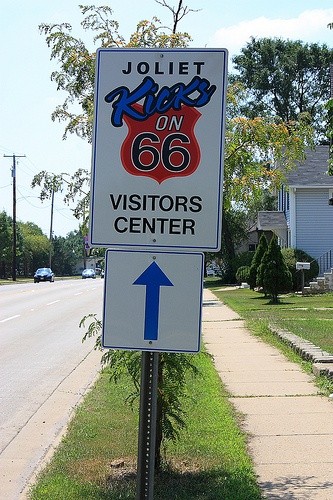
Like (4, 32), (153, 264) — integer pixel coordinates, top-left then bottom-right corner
(33, 268), (55, 284)
(82, 268), (105, 279)
